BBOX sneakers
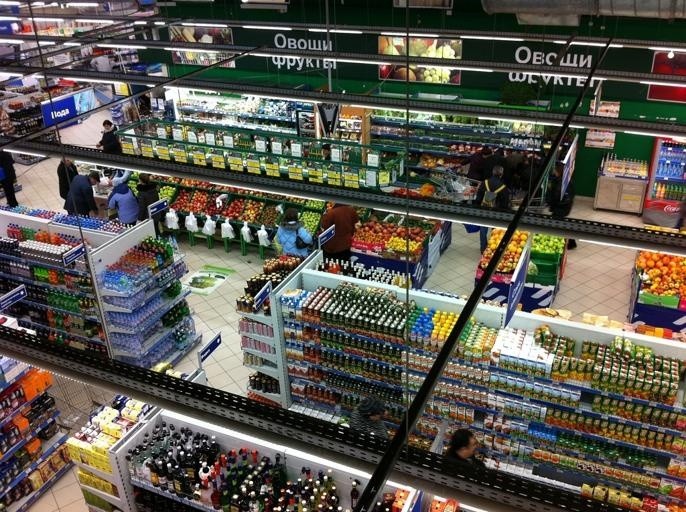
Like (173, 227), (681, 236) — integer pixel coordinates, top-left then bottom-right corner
(568, 239), (577, 250)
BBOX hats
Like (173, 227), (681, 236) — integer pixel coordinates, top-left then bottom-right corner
(89, 170), (100, 184)
(359, 397), (381, 413)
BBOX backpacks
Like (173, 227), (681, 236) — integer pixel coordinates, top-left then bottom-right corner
(481, 180), (505, 209)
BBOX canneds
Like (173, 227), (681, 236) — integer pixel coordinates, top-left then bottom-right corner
(236, 254), (417, 394)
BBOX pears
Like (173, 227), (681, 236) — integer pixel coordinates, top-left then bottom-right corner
(382, 37), (399, 55)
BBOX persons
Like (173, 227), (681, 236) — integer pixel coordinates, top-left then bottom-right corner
(443, 429), (486, 478)
(0, 147), (18, 207)
(90, 55), (117, 73)
(96, 120), (122, 154)
(138, 95), (150, 113)
(322, 144), (331, 160)
(276, 204), (359, 275)
(461, 146), (577, 255)
(107, 170), (161, 238)
(57, 157), (99, 218)
(350, 396), (389, 438)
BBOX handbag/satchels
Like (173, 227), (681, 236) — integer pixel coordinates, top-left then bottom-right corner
(464, 223), (481, 234)
(295, 237), (307, 250)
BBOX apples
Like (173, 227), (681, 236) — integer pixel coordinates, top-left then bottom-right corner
(531, 233), (565, 255)
(171, 188), (245, 220)
(362, 220), (426, 242)
(479, 229), (528, 273)
(380, 65), (391, 79)
(299, 199), (325, 236)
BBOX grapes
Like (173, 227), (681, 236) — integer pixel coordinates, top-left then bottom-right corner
(416, 68), (451, 83)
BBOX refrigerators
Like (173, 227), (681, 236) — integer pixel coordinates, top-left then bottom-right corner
(642, 136), (686, 228)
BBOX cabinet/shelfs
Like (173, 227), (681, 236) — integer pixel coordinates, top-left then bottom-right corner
(0, 0), (686, 512)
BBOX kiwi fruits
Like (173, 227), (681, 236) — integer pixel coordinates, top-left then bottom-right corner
(392, 68), (416, 81)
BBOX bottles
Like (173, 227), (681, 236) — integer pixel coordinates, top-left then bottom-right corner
(648, 148), (686, 199)
(0, 205), (196, 370)
(126, 421), (394, 512)
(236, 252), (685, 472)
(0, 387), (31, 512)
